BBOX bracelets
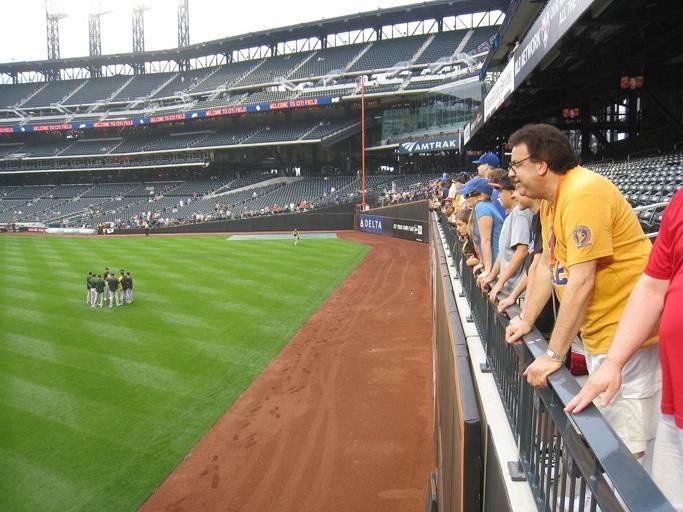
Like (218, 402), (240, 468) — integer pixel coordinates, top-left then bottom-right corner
(545, 349), (563, 360)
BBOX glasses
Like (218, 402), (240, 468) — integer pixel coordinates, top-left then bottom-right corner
(465, 193), (480, 199)
(509, 156), (532, 170)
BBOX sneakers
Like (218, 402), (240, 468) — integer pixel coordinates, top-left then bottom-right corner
(538, 437), (560, 482)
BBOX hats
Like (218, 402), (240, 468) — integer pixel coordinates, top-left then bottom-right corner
(459, 173), (514, 195)
(472, 152), (500, 167)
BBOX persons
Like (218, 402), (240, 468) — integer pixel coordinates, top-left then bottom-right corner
(143, 221), (149, 239)
(1, 46), (435, 232)
(292, 228), (299, 246)
(85, 266), (133, 309)
(436, 153), (574, 371)
(502, 124), (664, 482)
(563, 185), (682, 510)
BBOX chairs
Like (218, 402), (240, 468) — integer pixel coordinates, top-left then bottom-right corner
(581, 152), (683, 245)
